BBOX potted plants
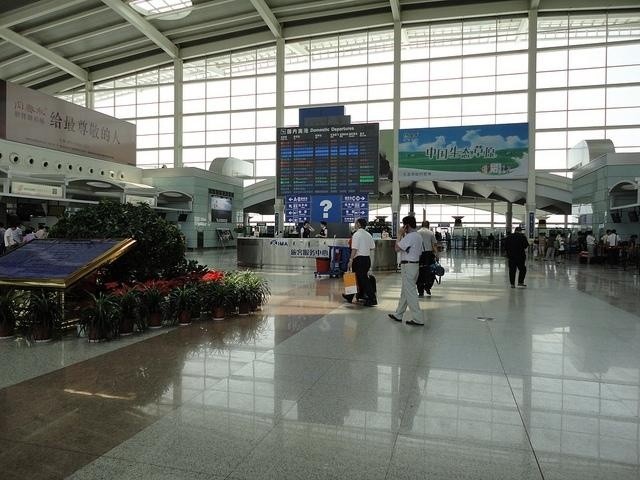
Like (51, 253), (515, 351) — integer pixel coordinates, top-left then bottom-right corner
(0, 259), (271, 343)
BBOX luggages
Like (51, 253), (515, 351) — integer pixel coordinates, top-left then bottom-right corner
(356, 274), (377, 302)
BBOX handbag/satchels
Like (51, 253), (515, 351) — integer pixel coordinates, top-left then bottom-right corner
(342, 271), (357, 296)
(432, 260), (445, 278)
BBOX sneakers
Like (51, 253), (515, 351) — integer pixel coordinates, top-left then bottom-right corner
(510, 283), (526, 288)
(388, 313), (402, 322)
(534, 257), (565, 265)
(405, 319), (424, 326)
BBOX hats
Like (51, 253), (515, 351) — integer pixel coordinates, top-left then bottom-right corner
(515, 226), (525, 232)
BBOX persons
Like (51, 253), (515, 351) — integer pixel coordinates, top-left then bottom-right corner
(300, 222), (315, 238)
(342, 218), (377, 306)
(473, 229), (637, 262)
(318, 221), (328, 237)
(0, 223), (49, 256)
(416, 221), (438, 296)
(433, 231), (452, 251)
(502, 227), (529, 288)
(388, 216), (425, 326)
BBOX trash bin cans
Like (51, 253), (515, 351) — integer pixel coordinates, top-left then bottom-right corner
(197, 232), (204, 248)
(579, 252), (588, 264)
(316, 257), (330, 273)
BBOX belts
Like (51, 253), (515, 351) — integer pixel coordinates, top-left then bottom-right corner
(399, 260), (419, 265)
